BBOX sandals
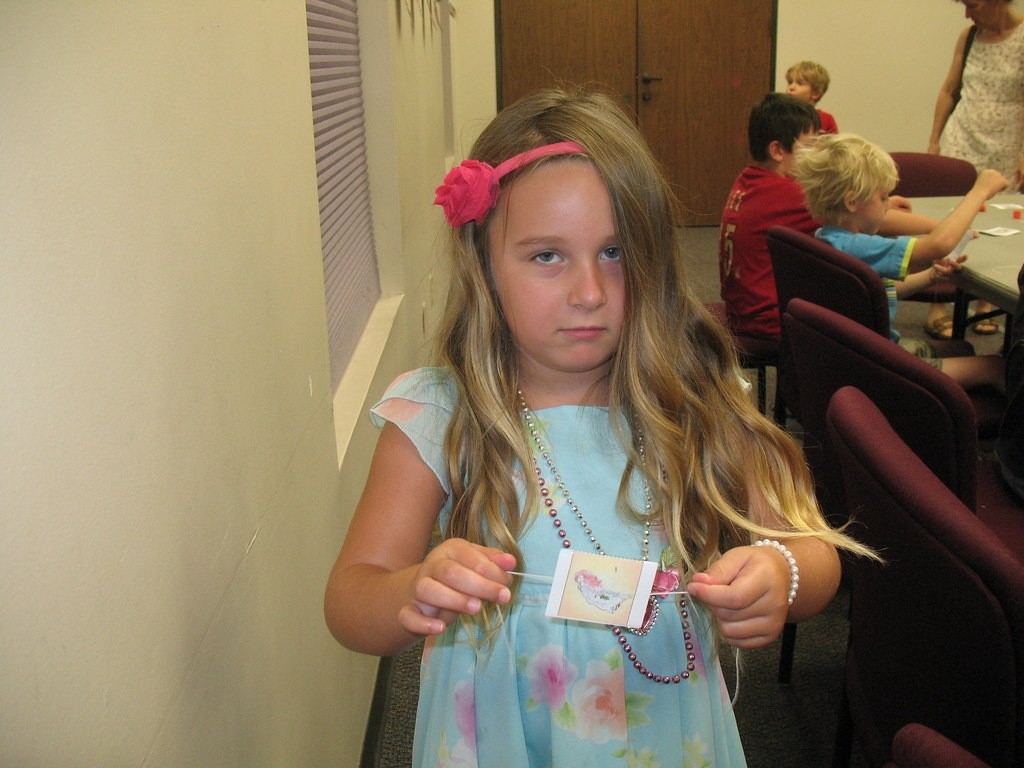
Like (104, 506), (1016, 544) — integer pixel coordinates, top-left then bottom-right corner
(973, 312), (1000, 335)
(924, 315), (954, 339)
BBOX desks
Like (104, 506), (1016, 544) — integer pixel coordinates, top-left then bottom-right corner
(905, 186), (1024, 352)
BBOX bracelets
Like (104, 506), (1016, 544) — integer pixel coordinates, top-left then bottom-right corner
(752, 538), (800, 606)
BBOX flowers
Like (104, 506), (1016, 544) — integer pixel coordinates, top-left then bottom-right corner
(433, 160), (500, 227)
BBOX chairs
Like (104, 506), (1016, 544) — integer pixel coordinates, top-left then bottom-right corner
(691, 149), (1024, 768)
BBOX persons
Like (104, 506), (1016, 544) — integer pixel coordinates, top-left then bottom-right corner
(323, 88), (841, 768)
(784, 61), (839, 135)
(718, 92), (912, 345)
(926, 0), (1024, 339)
(796, 134), (1009, 394)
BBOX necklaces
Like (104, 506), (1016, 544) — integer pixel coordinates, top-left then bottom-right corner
(517, 388), (695, 684)
